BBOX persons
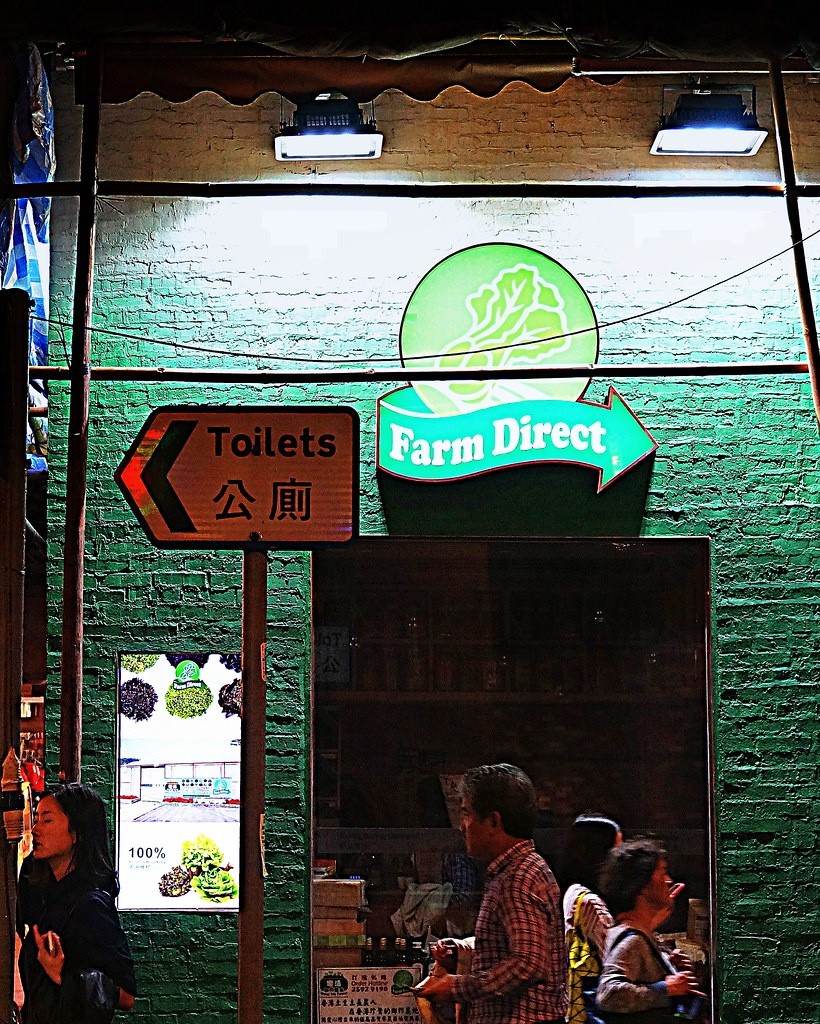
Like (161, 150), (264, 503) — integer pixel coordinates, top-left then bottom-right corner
(17, 785), (136, 1024)
(562, 814), (708, 1024)
(441, 848), (476, 908)
(414, 763), (569, 1024)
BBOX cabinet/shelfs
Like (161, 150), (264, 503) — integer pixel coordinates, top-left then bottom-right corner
(314, 539), (711, 906)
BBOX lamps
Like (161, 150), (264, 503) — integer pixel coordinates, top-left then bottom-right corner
(272, 93), (385, 161)
(650, 74), (767, 157)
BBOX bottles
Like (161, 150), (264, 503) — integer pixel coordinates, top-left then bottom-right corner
(364, 937), (436, 970)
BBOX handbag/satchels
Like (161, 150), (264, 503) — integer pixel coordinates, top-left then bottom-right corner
(579, 929), (708, 1024)
(566, 893), (601, 1024)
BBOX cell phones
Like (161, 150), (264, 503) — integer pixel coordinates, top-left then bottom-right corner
(47, 930), (56, 956)
